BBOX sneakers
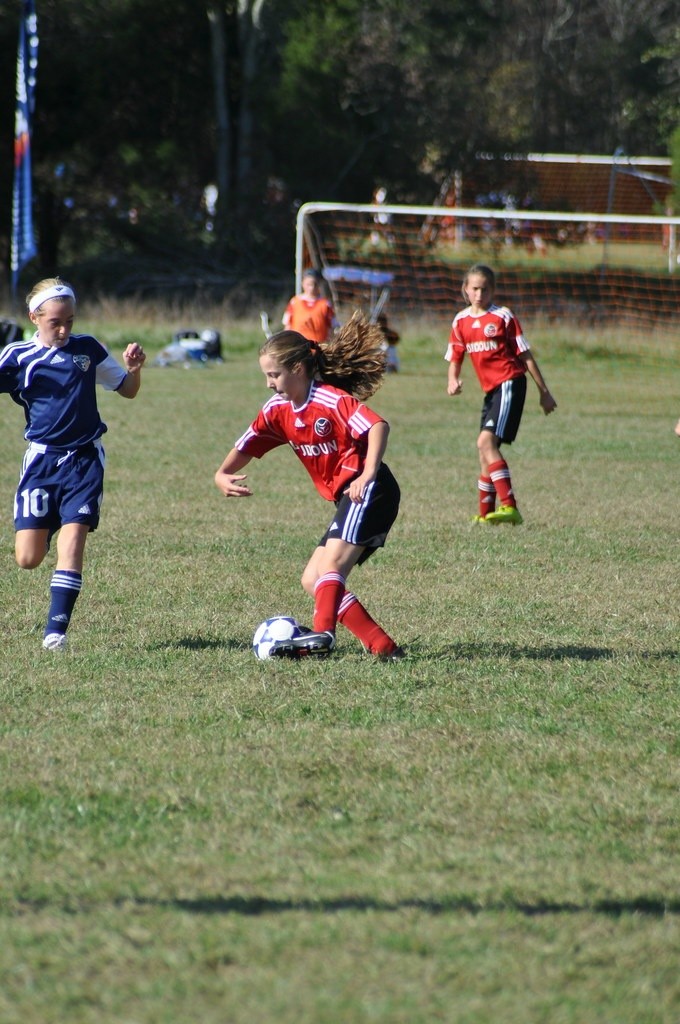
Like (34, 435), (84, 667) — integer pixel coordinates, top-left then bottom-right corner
(473, 515), (498, 525)
(43, 634), (66, 656)
(269, 631), (336, 658)
(485, 505), (522, 525)
(387, 648), (404, 662)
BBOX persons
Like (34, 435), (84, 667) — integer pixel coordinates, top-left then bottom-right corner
(0, 277), (147, 656)
(283, 268), (341, 352)
(375, 310), (400, 374)
(443, 265), (558, 529)
(213, 310), (406, 662)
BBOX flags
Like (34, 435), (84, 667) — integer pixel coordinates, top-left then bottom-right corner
(8, 11), (39, 275)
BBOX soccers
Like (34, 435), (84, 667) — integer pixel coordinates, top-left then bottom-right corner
(251, 616), (309, 663)
